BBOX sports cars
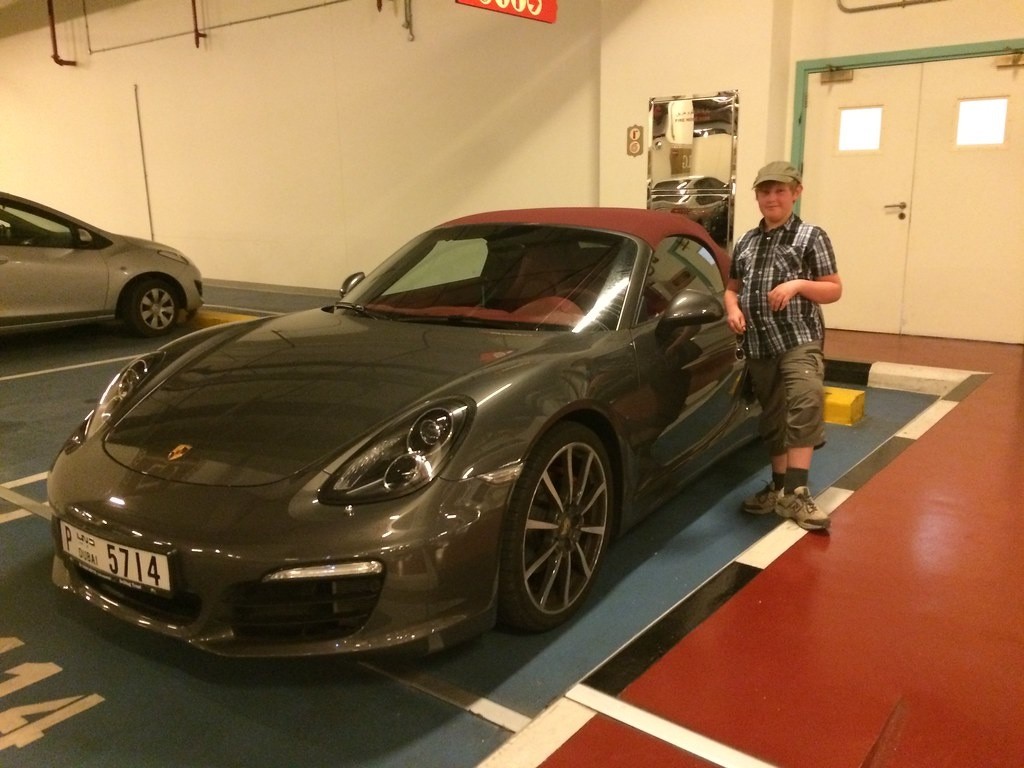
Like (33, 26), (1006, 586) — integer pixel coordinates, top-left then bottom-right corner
(46, 206), (770, 661)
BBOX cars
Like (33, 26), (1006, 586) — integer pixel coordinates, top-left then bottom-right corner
(1, 190), (206, 340)
(649, 177), (730, 224)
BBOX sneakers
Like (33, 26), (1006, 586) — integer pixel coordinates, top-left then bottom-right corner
(742, 480), (785, 515)
(775, 487), (830, 529)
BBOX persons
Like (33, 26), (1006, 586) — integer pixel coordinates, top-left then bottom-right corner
(724, 161), (843, 529)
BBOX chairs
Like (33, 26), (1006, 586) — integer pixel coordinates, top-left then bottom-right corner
(500, 241), (581, 309)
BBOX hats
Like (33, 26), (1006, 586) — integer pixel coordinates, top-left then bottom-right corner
(752, 161), (801, 190)
